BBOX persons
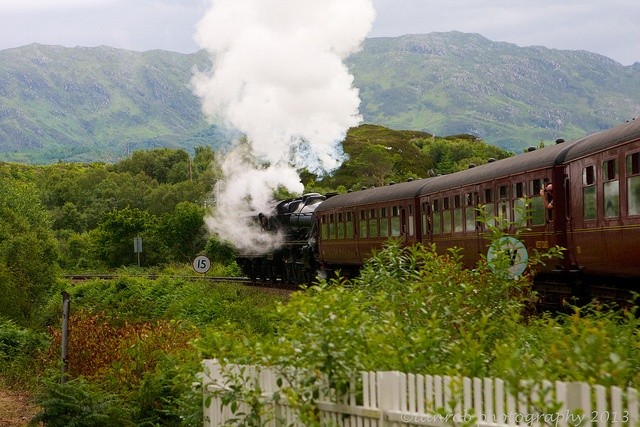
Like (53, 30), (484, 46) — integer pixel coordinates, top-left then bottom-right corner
(260, 215), (267, 225)
(427, 215), (433, 237)
(539, 182), (554, 209)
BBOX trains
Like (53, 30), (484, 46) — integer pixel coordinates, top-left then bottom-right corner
(235, 117), (640, 314)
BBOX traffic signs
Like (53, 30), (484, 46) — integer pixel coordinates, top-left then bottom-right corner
(193, 255), (210, 273)
